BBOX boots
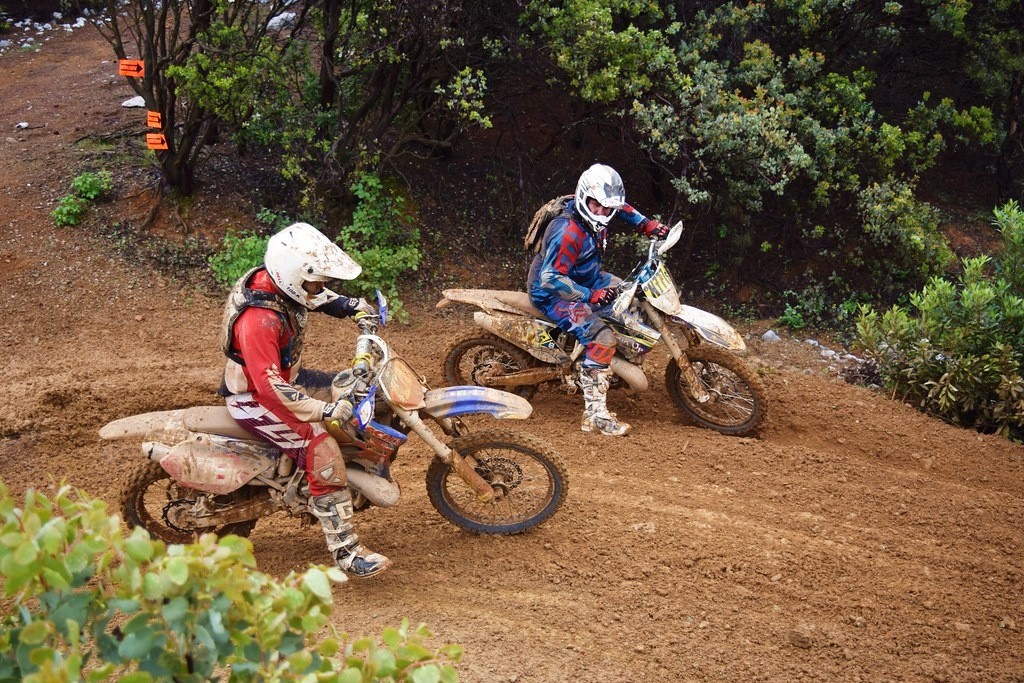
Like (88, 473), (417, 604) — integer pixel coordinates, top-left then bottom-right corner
(311, 489), (391, 578)
(580, 366), (632, 435)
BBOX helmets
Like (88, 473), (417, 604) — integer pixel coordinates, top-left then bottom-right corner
(575, 163), (626, 231)
(264, 223), (363, 310)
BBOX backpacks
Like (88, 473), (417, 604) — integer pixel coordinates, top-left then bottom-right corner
(524, 194), (573, 256)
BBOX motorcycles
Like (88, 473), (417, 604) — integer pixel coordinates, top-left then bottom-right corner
(98, 291), (571, 549)
(435, 220), (770, 436)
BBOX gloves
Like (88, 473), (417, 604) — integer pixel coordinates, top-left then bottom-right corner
(636, 217), (670, 241)
(322, 400), (353, 421)
(590, 286), (617, 306)
(342, 296), (375, 322)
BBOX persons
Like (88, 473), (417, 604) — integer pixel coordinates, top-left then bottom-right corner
(220, 222), (393, 578)
(528, 164), (671, 437)
